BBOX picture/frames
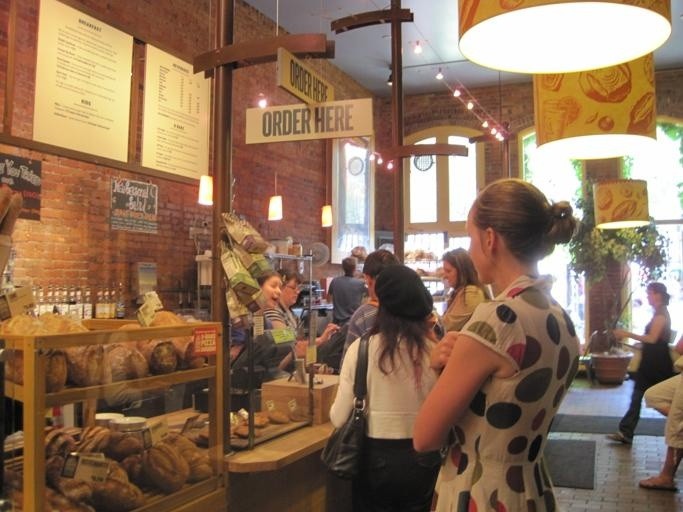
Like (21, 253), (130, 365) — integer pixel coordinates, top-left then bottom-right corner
(326, 115), (376, 265)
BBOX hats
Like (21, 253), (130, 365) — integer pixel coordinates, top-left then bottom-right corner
(374, 265), (433, 318)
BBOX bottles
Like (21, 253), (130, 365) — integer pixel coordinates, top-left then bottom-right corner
(286, 235), (293, 255)
(29, 282), (125, 321)
(107, 414), (153, 456)
(292, 242), (302, 257)
(94, 412), (124, 428)
(296, 281), (321, 308)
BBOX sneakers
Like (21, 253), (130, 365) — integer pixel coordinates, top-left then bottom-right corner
(640, 476), (673, 493)
(605, 431), (632, 446)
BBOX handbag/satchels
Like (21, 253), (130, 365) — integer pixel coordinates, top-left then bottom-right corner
(318, 397), (369, 481)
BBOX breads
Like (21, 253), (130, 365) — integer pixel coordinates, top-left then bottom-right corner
(1, 312), (210, 392)
(2, 414), (212, 512)
(230, 408), (304, 451)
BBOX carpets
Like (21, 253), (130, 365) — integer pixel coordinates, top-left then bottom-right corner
(550, 415), (667, 435)
(542, 440), (596, 489)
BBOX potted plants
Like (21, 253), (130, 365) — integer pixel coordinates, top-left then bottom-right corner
(570, 175), (671, 384)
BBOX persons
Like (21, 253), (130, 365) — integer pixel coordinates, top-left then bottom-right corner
(641, 336), (683, 490)
(339, 250), (400, 374)
(326, 256), (368, 327)
(329, 264), (443, 511)
(607, 282), (675, 444)
(351, 243), (368, 276)
(434, 247), (489, 333)
(413, 177), (581, 512)
(229, 268), (298, 412)
(276, 269), (343, 371)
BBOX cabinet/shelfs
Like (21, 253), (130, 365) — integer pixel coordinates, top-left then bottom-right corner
(0, 318), (227, 512)
(195, 248), (312, 395)
(401, 257), (450, 320)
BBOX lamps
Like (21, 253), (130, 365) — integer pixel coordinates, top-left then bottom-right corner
(197, 78), (214, 206)
(320, 140), (333, 229)
(459, 2), (674, 75)
(592, 158), (651, 231)
(533, 44), (658, 160)
(267, 142), (284, 219)
